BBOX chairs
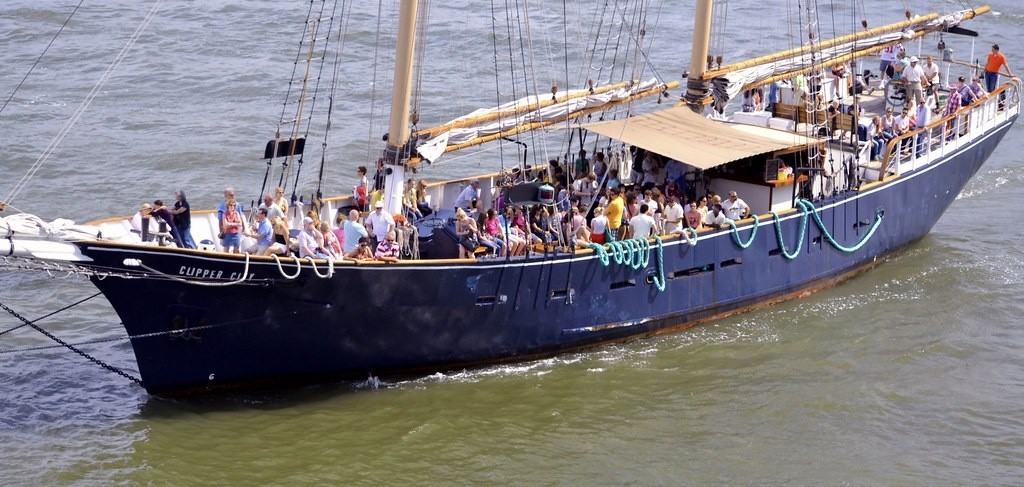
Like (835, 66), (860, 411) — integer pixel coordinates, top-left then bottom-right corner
(765, 104), (856, 146)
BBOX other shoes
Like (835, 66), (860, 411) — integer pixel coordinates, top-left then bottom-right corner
(874, 154), (883, 161)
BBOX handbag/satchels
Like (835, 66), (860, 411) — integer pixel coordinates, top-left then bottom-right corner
(218, 231), (226, 239)
(605, 228), (613, 243)
(460, 234), (479, 253)
(574, 181), (583, 209)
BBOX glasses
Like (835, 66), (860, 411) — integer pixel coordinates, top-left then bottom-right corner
(422, 186), (426, 189)
(376, 208), (382, 210)
(357, 170), (362, 172)
(609, 193), (617, 196)
(708, 196), (711, 199)
(586, 182), (588, 188)
(387, 239), (394, 242)
(361, 244), (368, 247)
(229, 204), (236, 207)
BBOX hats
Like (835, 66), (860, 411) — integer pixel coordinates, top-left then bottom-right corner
(949, 83), (958, 90)
(609, 169), (618, 177)
(375, 201), (384, 208)
(910, 56), (919, 63)
(139, 203), (152, 211)
(470, 179), (482, 184)
(302, 217), (314, 226)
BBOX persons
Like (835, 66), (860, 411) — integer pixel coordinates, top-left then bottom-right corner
(223, 199), (242, 253)
(376, 231), (400, 261)
(629, 205), (657, 239)
(344, 210), (368, 254)
(352, 166), (370, 213)
(720, 191), (750, 220)
(589, 207), (610, 245)
(454, 179), (480, 214)
(129, 40), (1017, 261)
(901, 56), (930, 107)
(659, 197), (683, 234)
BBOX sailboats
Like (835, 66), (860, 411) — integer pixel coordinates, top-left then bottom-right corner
(0, 1), (1024, 397)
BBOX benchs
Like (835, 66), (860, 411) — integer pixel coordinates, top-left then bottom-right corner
(504, 182), (557, 213)
(473, 239), (581, 254)
(849, 69), (872, 95)
